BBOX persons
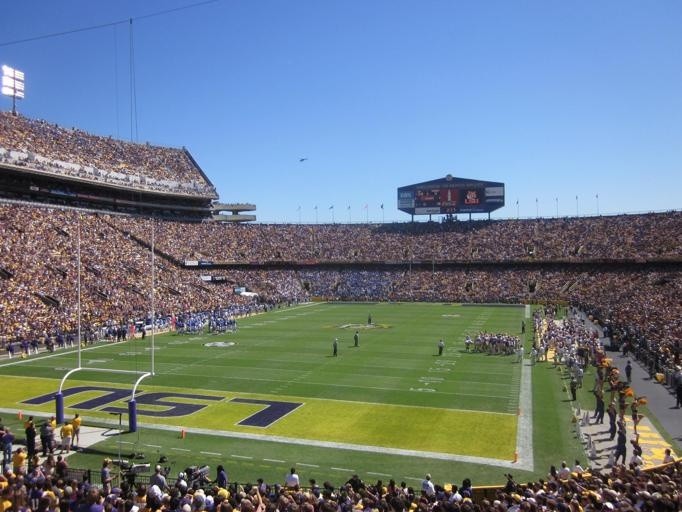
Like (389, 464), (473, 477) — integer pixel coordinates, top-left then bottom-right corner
(0, 212), (682, 512)
(0, 108), (218, 200)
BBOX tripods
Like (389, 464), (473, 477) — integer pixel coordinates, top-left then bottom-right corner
(126, 477), (137, 493)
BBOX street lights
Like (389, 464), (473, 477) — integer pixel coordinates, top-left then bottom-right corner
(1, 63), (26, 114)
